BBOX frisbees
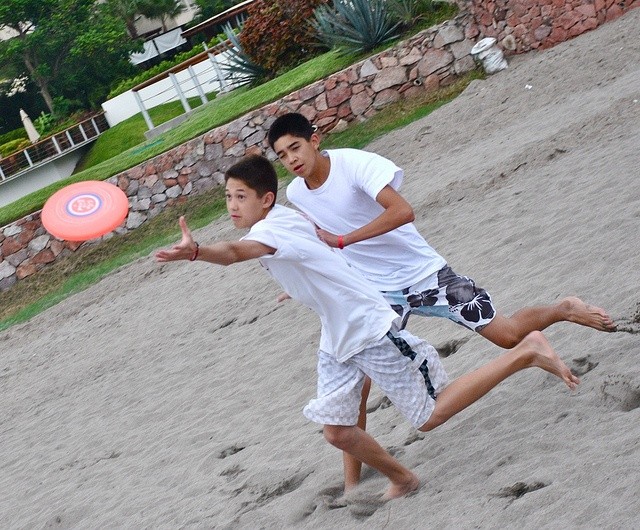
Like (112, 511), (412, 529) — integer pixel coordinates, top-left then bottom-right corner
(40, 180), (128, 242)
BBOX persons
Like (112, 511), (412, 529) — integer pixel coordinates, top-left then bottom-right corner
(268, 112), (618, 495)
(154, 153), (581, 501)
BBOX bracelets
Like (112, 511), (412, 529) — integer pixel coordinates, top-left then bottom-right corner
(338, 235), (344, 249)
(190, 242), (199, 261)
